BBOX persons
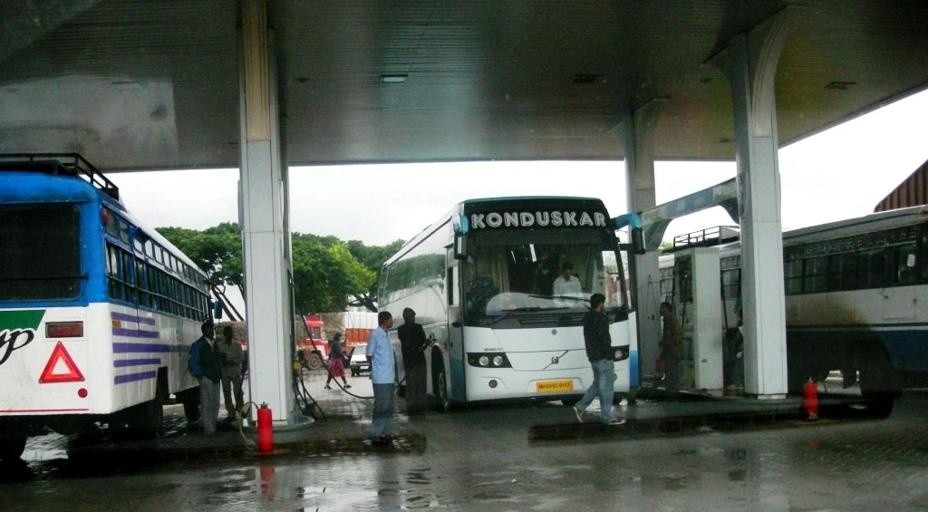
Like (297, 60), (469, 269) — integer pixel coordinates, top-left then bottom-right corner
(724, 309), (744, 391)
(397, 307), (428, 415)
(366, 311), (396, 446)
(218, 325), (245, 421)
(552, 261), (582, 295)
(571, 293), (626, 425)
(656, 302), (685, 392)
(188, 321), (226, 438)
(323, 332), (353, 389)
(464, 273), (500, 311)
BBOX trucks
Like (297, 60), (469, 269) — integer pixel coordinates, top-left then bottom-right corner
(216, 312), (333, 371)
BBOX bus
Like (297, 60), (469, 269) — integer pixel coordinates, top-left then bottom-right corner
(616, 205), (926, 412)
(0, 153), (222, 462)
(378, 195), (646, 413)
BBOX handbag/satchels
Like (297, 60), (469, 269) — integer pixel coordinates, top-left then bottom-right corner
(656, 358), (667, 371)
(672, 342), (687, 360)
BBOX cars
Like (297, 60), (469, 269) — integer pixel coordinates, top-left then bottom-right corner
(350, 343), (371, 376)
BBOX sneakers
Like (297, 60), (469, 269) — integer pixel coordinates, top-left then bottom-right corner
(407, 405), (426, 417)
(344, 385), (351, 388)
(573, 405), (585, 424)
(603, 417), (626, 426)
(224, 414), (236, 424)
(371, 437), (389, 448)
(383, 434), (399, 442)
(327, 385), (331, 390)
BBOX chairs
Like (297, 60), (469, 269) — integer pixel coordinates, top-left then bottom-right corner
(470, 275), (499, 311)
